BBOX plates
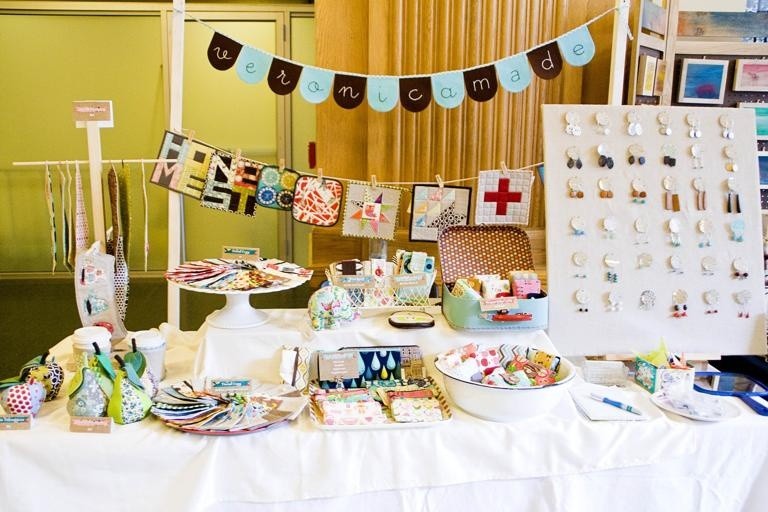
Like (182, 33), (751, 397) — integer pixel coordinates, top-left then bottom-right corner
(651, 388), (745, 421)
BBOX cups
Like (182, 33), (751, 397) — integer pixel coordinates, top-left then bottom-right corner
(127, 330), (166, 382)
(72, 326), (111, 371)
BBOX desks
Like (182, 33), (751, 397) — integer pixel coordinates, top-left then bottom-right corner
(1, 302), (768, 510)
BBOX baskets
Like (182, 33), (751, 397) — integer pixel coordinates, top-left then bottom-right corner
(325, 261), (440, 311)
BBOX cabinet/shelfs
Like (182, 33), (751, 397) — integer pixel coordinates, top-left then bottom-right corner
(624, 1), (767, 259)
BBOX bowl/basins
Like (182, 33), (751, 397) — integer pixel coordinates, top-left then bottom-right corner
(434, 342), (577, 422)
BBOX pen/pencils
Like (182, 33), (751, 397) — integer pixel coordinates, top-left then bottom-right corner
(590, 393), (641, 415)
